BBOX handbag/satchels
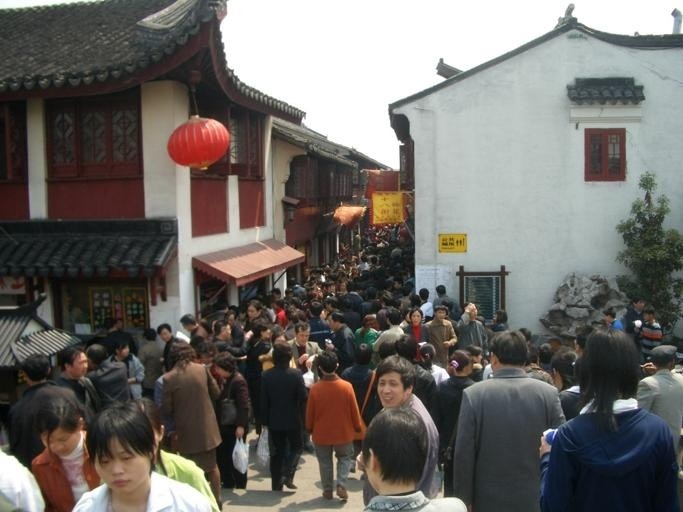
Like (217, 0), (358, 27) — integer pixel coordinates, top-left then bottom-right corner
(256, 428), (269, 468)
(438, 447), (453, 472)
(221, 376), (253, 425)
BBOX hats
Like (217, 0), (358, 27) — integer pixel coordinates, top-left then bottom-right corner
(650, 345), (678, 360)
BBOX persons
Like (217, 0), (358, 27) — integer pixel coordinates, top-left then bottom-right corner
(161, 341), (222, 510)
(634, 310), (664, 361)
(159, 323), (180, 372)
(30, 387), (103, 512)
(340, 345), (379, 480)
(179, 291), (292, 346)
(50, 350), (103, 414)
(86, 335), (107, 366)
(154, 355), (173, 443)
(489, 310), (554, 376)
(432, 354), (475, 498)
(287, 299), (362, 338)
(1, 448), (48, 512)
(572, 325), (596, 361)
(258, 344), (308, 493)
(636, 345), (683, 511)
(538, 323), (683, 512)
(550, 348), (592, 419)
(304, 354), (363, 498)
(453, 330), (567, 511)
(271, 223), (413, 309)
(108, 346), (147, 399)
(5, 353), (81, 467)
(213, 352), (251, 488)
(623, 296), (647, 333)
(602, 307), (625, 331)
(137, 328), (164, 399)
(123, 397), (220, 511)
(355, 353), (440, 499)
(69, 406), (215, 512)
(361, 405), (466, 510)
(85, 343), (135, 414)
(102, 318), (136, 355)
(356, 284), (490, 378)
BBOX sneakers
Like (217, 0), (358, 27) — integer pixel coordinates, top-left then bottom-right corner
(283, 481), (297, 489)
(336, 486), (348, 499)
(323, 491), (333, 499)
